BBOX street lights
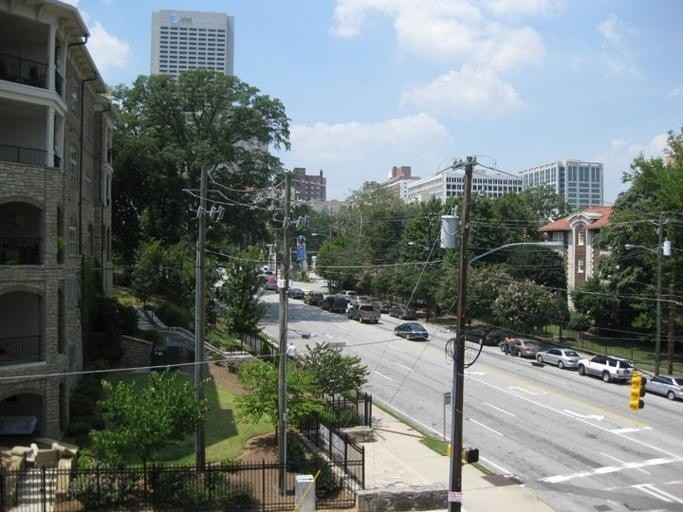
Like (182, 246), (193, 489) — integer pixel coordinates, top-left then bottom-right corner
(451, 240), (566, 510)
(406, 240), (429, 322)
(623, 244), (660, 376)
(311, 232), (332, 255)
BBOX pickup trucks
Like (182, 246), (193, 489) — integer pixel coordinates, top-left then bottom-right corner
(347, 304), (380, 324)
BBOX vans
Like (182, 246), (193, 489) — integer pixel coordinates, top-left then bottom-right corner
(321, 296), (347, 313)
(576, 355), (633, 382)
(336, 290), (355, 301)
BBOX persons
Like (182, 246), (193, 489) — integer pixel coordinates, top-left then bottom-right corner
(504, 337), (510, 356)
(286, 342), (296, 359)
(510, 338), (516, 356)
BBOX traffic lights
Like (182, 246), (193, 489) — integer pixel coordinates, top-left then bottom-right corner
(630, 375), (646, 409)
(466, 447), (479, 464)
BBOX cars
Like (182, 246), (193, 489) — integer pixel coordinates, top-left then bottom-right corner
(645, 373), (682, 399)
(499, 338), (539, 358)
(395, 322), (427, 341)
(389, 306), (415, 319)
(351, 296), (370, 305)
(463, 326), (499, 346)
(535, 347), (580, 368)
(255, 265), (324, 306)
(371, 301), (390, 313)
